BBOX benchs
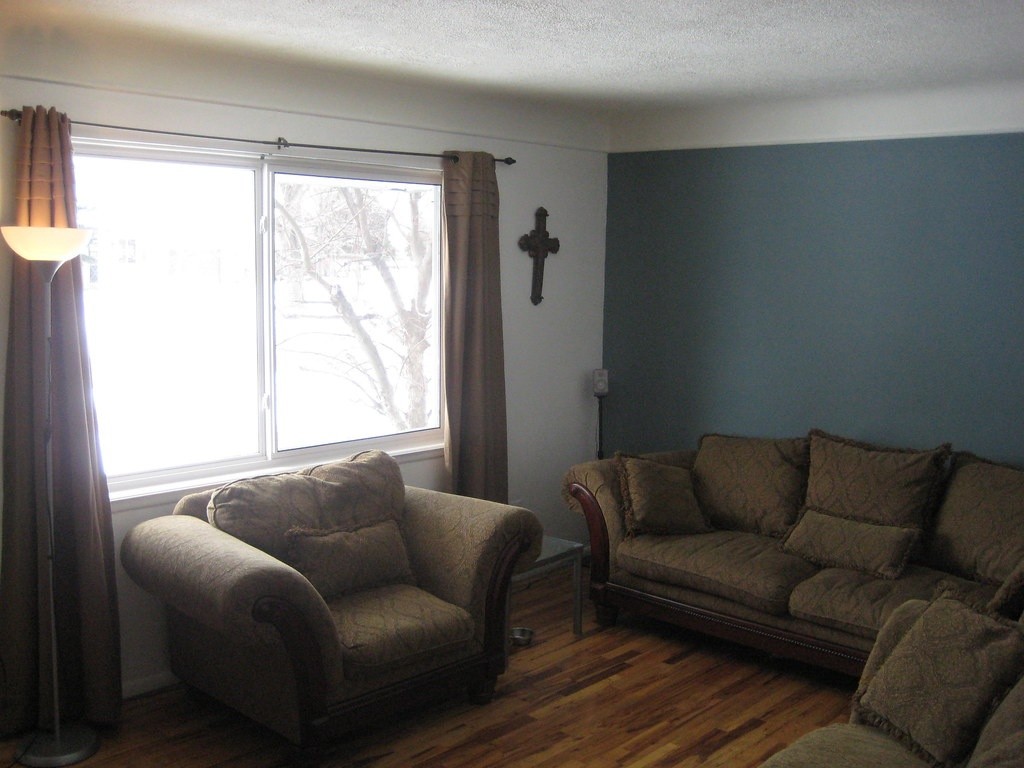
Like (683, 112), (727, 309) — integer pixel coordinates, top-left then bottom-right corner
(559, 452), (1024, 673)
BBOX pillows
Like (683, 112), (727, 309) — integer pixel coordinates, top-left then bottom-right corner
(803, 428), (953, 562)
(855, 581), (1024, 768)
(925, 451), (1024, 584)
(780, 504), (922, 580)
(614, 450), (716, 538)
(212, 450), (407, 599)
(690, 433), (808, 536)
(287, 518), (414, 600)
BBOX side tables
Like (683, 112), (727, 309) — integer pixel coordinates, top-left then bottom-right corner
(504, 536), (585, 665)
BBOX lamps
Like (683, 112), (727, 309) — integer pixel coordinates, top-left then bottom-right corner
(0, 226), (101, 768)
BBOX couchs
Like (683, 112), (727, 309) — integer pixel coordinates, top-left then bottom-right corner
(124, 448), (546, 768)
(762, 597), (1024, 768)
